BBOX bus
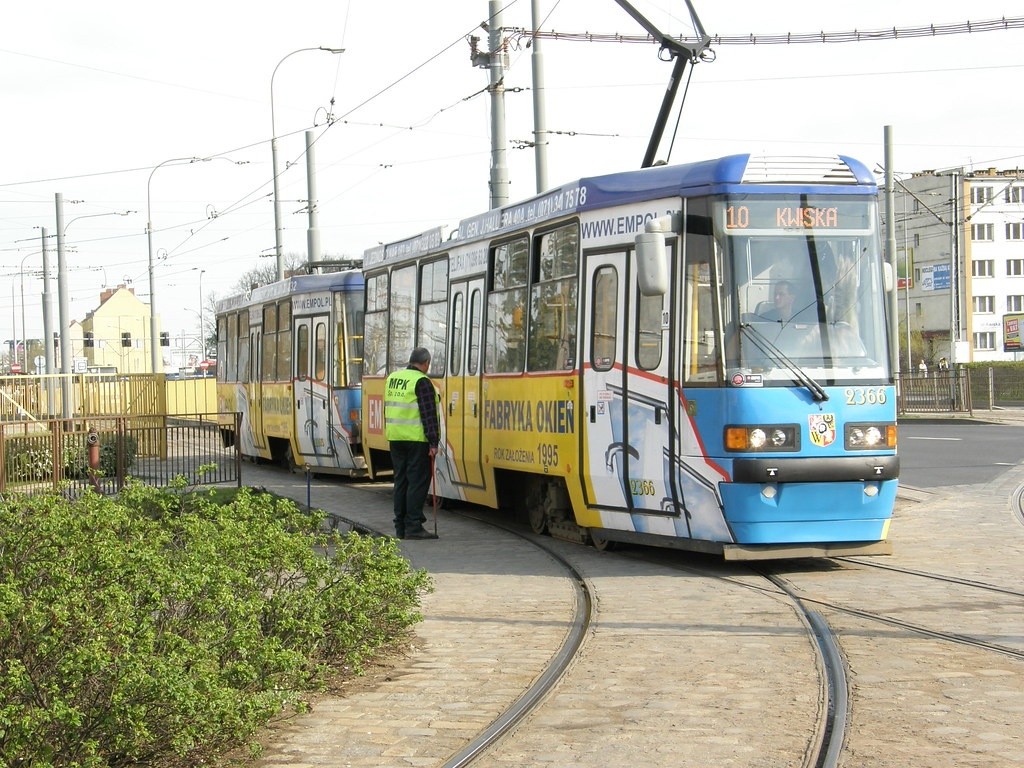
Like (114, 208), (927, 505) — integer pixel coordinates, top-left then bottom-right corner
(213, 257), (371, 481)
(355, 1), (903, 568)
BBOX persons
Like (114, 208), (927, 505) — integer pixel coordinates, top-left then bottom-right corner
(383, 347), (441, 541)
(757, 280), (805, 323)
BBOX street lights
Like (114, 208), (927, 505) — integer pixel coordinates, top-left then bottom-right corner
(873, 168), (1024, 409)
(268, 46), (349, 280)
(147, 155), (213, 374)
(11, 247), (78, 375)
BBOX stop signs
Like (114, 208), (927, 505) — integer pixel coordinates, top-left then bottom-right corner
(11, 363), (21, 373)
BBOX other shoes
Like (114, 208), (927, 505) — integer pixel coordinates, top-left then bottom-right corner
(394, 527), (439, 541)
